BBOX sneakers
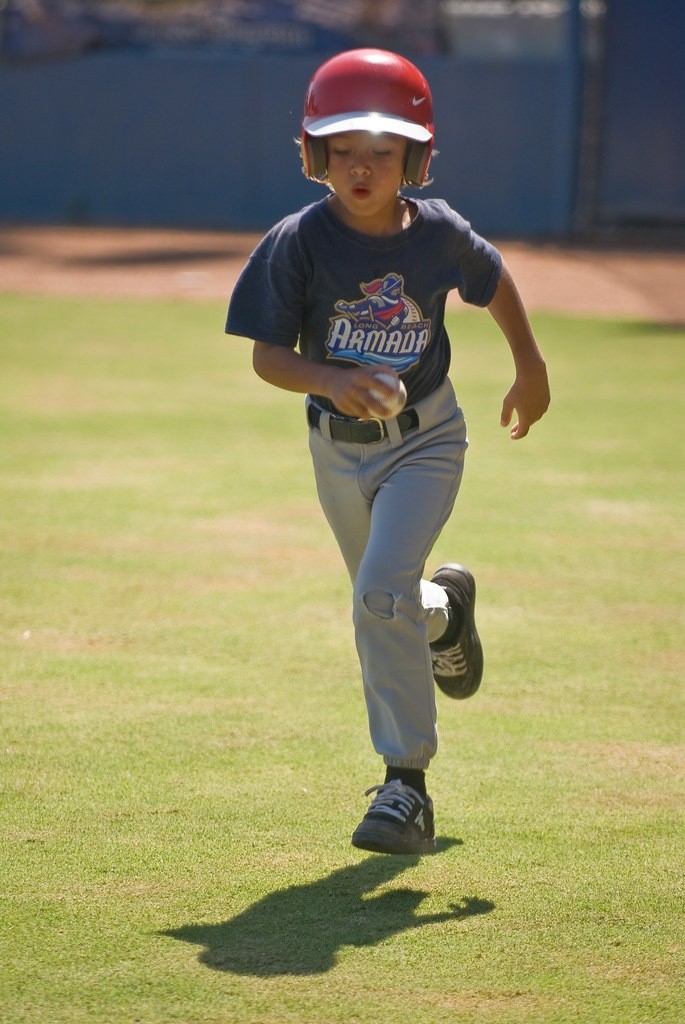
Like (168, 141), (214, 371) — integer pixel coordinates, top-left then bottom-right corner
(428, 562), (484, 699)
(352, 778), (437, 852)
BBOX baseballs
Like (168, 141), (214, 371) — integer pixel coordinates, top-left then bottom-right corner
(366, 372), (409, 420)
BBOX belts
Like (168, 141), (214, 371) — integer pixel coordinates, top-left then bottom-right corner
(309, 403), (418, 443)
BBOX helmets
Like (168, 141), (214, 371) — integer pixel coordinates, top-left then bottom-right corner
(301, 47), (434, 186)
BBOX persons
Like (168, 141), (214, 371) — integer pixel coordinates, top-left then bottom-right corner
(225, 47), (551, 854)
